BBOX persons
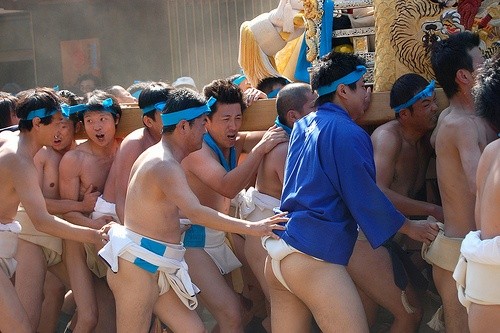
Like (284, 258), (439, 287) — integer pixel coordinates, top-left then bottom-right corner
(346, 73), (444, 333)
(421, 30), (485, 333)
(452, 57), (500, 333)
(261, 50), (370, 333)
(0, 75), (321, 333)
